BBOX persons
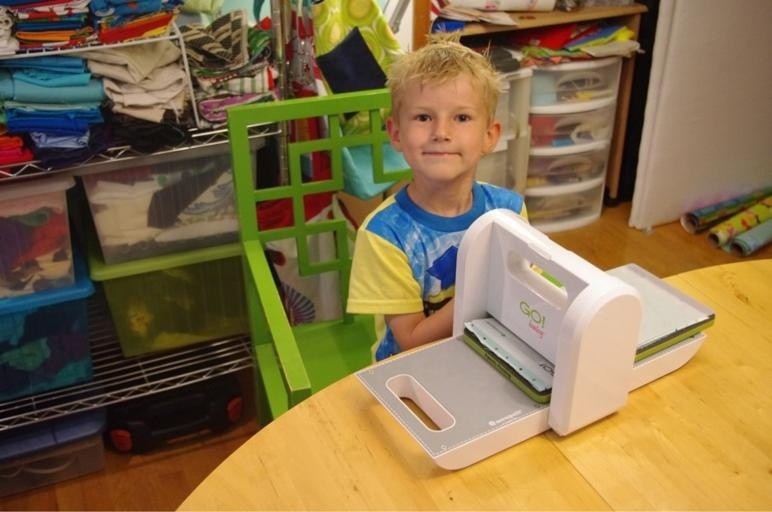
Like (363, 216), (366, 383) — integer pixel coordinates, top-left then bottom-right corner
(344, 30), (531, 359)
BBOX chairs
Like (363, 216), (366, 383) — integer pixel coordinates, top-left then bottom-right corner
(226, 89), (413, 428)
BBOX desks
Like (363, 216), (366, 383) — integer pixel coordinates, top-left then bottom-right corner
(174, 257), (772, 512)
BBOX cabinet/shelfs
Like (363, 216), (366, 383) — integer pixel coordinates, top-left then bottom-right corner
(1, 0), (288, 436)
(414, 0), (647, 208)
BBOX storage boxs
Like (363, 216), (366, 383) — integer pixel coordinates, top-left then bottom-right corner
(1, 139), (78, 299)
(473, 46), (623, 234)
(83, 234), (265, 356)
(71, 139), (265, 266)
(0, 272), (98, 405)
